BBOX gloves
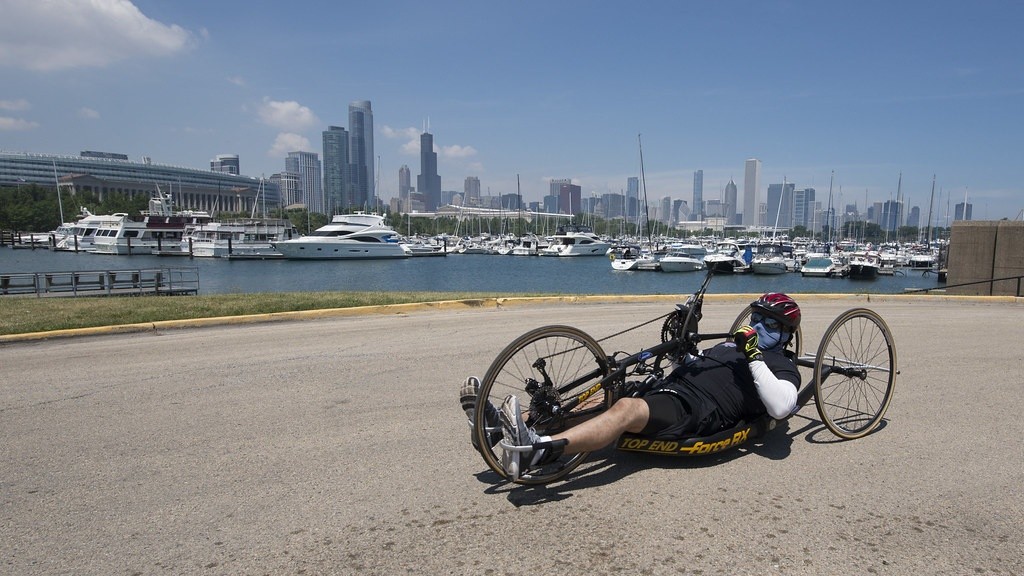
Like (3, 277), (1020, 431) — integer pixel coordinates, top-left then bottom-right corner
(734, 324), (762, 359)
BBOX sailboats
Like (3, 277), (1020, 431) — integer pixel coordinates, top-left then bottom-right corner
(14, 131), (950, 278)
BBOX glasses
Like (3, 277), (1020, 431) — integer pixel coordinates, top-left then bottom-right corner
(751, 312), (789, 330)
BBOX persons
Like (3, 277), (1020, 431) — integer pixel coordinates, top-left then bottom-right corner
(459, 293), (802, 482)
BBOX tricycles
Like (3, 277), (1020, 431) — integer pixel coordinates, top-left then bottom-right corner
(473, 261), (900, 486)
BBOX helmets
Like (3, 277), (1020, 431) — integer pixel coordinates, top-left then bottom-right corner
(750, 291), (801, 334)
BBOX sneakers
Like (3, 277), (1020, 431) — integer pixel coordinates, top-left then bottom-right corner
(498, 395), (543, 481)
(460, 376), (505, 452)
